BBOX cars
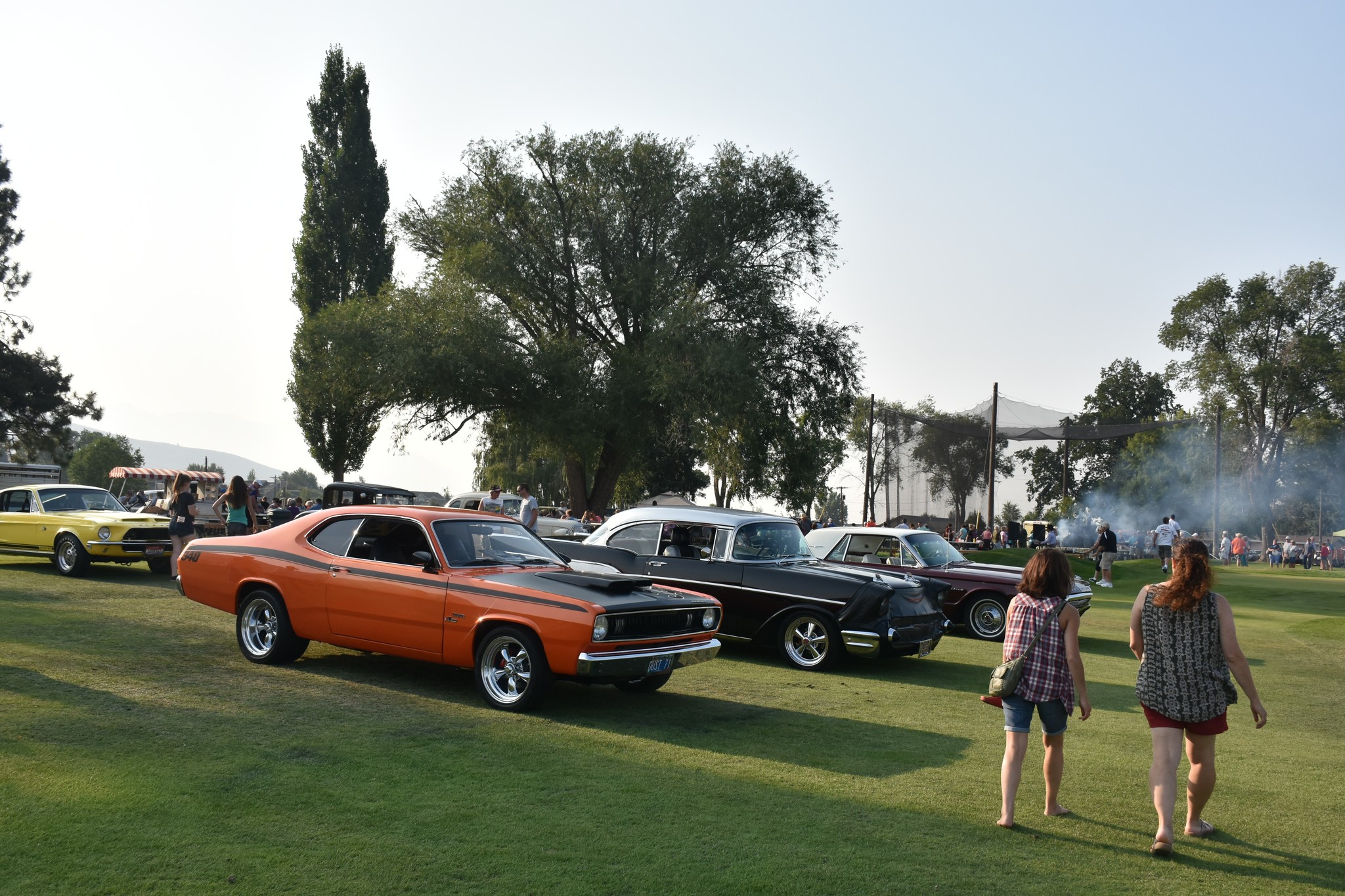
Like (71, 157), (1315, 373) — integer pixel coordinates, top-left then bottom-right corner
(175, 502), (728, 708)
(800, 527), (1094, 642)
(540, 507), (955, 673)
(0, 483), (173, 578)
(446, 491), (589, 538)
(294, 481), (417, 520)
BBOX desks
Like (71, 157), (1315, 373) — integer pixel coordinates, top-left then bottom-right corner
(1117, 549), (1130, 560)
(590, 523), (660, 531)
(880, 548), (897, 552)
(949, 541), (984, 550)
(221, 513), (269, 516)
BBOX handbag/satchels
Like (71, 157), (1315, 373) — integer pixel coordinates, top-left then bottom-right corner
(988, 657), (1024, 696)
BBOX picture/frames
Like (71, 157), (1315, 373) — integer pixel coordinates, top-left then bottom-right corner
(478, 549), (525, 563)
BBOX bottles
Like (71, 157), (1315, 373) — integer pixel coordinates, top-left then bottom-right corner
(973, 538), (975, 542)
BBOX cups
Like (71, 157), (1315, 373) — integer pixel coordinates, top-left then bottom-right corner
(960, 547), (962, 550)
(1093, 556), (1096, 560)
(585, 520), (588, 524)
(600, 520), (603, 523)
(1073, 550), (1074, 553)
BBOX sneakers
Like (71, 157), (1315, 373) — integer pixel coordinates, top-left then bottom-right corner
(1089, 577), (1112, 587)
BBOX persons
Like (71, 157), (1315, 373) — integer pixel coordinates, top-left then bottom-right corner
(167, 473), (201, 580)
(796, 512), (1058, 554)
(118, 489), (165, 514)
(477, 486), (505, 559)
(990, 546), (1094, 829)
(1129, 539), (1267, 854)
(653, 501), (656, 507)
(545, 499), (623, 539)
(341, 498), (353, 506)
(1078, 514), (1345, 588)
(212, 475), (263, 536)
(517, 484), (538, 549)
(260, 496), (324, 523)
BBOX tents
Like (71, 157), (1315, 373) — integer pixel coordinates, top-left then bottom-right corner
(629, 490), (698, 507)
(1331, 529), (1345, 545)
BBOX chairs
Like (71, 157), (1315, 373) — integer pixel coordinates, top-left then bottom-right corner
(861, 554), (881, 563)
(665, 528), (674, 538)
(886, 557), (906, 565)
(639, 530), (658, 539)
(1039, 540), (1057, 550)
(750, 535), (774, 549)
(670, 526), (701, 558)
(583, 525), (591, 533)
(439, 534), (470, 561)
(709, 528), (738, 559)
(369, 538), (408, 564)
(693, 527), (712, 546)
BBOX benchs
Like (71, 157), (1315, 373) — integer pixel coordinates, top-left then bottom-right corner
(156, 499), (170, 514)
(832, 554), (863, 562)
(957, 550), (984, 553)
(608, 539), (671, 556)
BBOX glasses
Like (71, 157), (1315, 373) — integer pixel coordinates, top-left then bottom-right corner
(1097, 530), (1099, 531)
(277, 503), (280, 506)
(518, 489), (523, 492)
(296, 501), (301, 503)
(1101, 526), (1102, 527)
(494, 491), (501, 494)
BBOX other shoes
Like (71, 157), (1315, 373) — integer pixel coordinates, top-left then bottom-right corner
(170, 576), (176, 581)
(1163, 567), (1167, 573)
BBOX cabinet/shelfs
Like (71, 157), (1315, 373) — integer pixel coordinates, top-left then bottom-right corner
(204, 481), (217, 501)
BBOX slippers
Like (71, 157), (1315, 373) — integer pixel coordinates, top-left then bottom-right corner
(1183, 818), (1213, 836)
(1150, 839), (1173, 856)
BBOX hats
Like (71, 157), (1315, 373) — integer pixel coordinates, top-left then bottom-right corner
(1272, 550), (1279, 556)
(490, 485), (503, 492)
(1286, 536), (1290, 539)
(541, 509), (548, 513)
(1171, 514), (1175, 518)
(254, 479), (263, 486)
(800, 513), (833, 522)
(1044, 524), (1056, 530)
(1192, 533), (1198, 536)
(864, 522), (867, 524)
(220, 488), (226, 493)
(127, 491), (132, 495)
(1325, 539), (1329, 541)
(1306, 538), (1311, 541)
(138, 490), (144, 493)
(903, 518), (907, 522)
